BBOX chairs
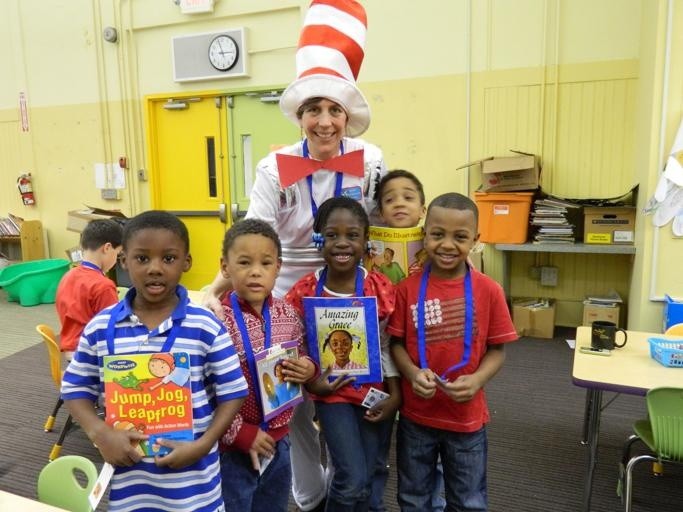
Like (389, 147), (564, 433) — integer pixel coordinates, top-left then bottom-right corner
(614, 387), (682, 511)
(37, 326), (101, 462)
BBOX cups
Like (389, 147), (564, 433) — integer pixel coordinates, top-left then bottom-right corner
(591, 320), (627, 350)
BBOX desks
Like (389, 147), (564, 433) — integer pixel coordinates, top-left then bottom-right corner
(495, 238), (636, 317)
(569, 327), (683, 511)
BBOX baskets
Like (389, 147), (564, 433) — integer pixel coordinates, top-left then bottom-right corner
(648, 337), (683, 367)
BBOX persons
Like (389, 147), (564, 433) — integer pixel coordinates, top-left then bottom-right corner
(207, 218), (323, 512)
(408, 247), (429, 277)
(377, 247), (407, 286)
(320, 329), (367, 370)
(269, 356), (299, 409)
(59, 210), (251, 512)
(200, 75), (389, 512)
(361, 169), (451, 512)
(385, 191), (520, 512)
(283, 196), (404, 511)
(55, 217), (127, 363)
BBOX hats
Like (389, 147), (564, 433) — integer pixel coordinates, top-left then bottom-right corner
(278, 1), (370, 137)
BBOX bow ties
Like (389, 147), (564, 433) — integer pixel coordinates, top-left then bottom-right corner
(276, 149), (363, 188)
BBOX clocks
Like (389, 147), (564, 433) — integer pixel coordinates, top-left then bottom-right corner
(208, 35), (237, 72)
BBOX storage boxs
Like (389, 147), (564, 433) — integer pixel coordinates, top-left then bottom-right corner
(582, 204), (636, 243)
(510, 295), (620, 339)
(455, 147), (541, 244)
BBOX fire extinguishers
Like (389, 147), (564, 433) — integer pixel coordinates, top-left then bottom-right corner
(16, 172), (35, 205)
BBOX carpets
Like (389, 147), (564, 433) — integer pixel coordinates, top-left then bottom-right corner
(0, 335), (683, 510)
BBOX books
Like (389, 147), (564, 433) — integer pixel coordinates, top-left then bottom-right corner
(513, 296), (554, 309)
(529, 198), (582, 245)
(582, 292), (624, 309)
(101, 351), (197, 463)
(362, 223), (430, 287)
(253, 341), (306, 422)
(300, 295), (385, 388)
(0, 212), (25, 236)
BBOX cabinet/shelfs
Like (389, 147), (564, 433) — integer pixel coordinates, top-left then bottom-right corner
(0, 219), (46, 272)
